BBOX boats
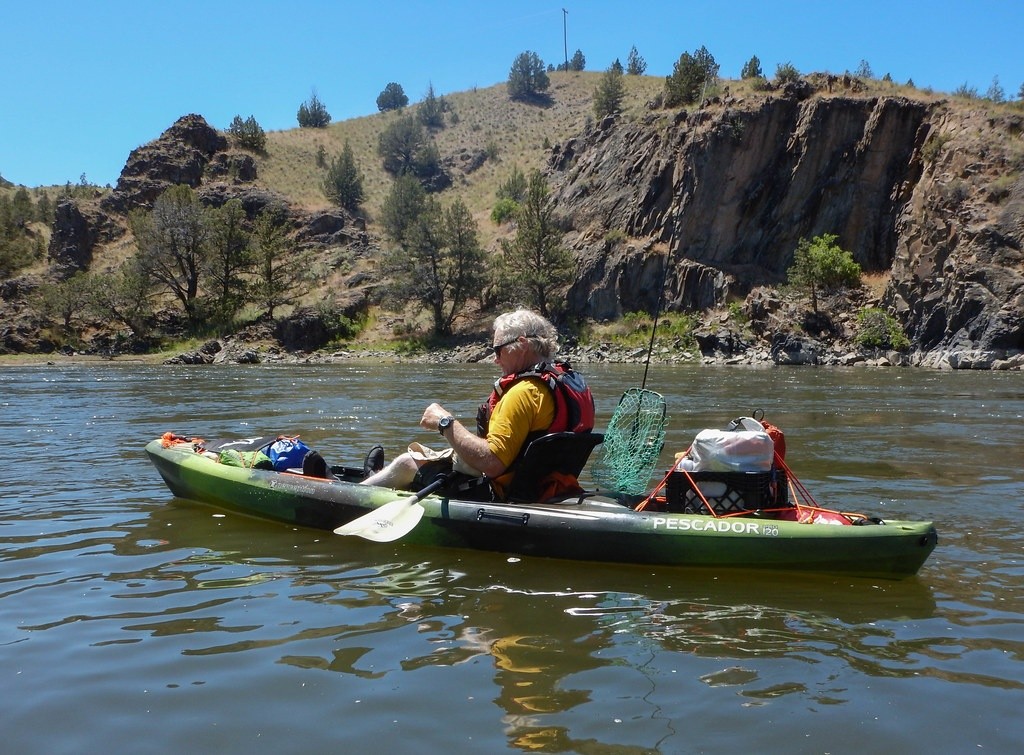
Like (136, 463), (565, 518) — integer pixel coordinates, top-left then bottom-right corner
(146, 432), (938, 582)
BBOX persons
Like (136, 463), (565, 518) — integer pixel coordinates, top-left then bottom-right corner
(301, 309), (595, 503)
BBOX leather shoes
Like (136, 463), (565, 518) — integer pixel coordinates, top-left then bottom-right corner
(301, 450), (334, 480)
(363, 446), (385, 479)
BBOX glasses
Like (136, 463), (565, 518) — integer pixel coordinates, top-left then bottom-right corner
(494, 335), (538, 357)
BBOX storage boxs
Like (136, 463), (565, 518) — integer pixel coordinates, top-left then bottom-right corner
(663, 469), (789, 519)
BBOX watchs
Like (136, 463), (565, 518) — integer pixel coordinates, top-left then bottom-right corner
(437, 416), (456, 437)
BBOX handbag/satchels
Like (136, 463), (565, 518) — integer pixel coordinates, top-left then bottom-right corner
(677, 428), (774, 469)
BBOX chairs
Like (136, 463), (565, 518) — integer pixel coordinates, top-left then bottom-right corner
(437, 431), (605, 501)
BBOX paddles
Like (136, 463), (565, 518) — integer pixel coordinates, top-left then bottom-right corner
(330, 469), (461, 545)
